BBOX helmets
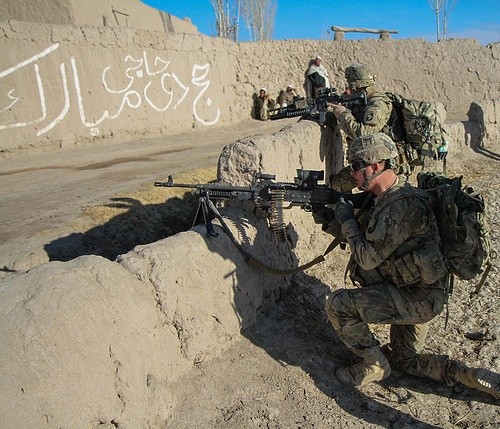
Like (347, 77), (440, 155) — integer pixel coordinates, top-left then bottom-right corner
(344, 63), (376, 91)
(348, 132), (399, 166)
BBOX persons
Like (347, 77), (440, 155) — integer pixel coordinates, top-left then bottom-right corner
(312, 133), (500, 397)
(306, 56), (330, 99)
(256, 88), (280, 120)
(325, 63), (415, 191)
(281, 85), (298, 109)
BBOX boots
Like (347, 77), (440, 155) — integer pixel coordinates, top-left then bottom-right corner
(336, 345), (392, 386)
(452, 361), (500, 401)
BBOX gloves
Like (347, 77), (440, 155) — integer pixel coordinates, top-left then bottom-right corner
(301, 203), (334, 224)
(334, 194), (354, 223)
(326, 102), (347, 118)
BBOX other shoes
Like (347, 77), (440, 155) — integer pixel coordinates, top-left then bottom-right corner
(261, 116), (267, 121)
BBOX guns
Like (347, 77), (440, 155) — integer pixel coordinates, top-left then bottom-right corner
(268, 88), (364, 125)
(155, 175), (369, 236)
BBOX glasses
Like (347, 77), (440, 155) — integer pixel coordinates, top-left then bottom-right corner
(349, 159), (386, 173)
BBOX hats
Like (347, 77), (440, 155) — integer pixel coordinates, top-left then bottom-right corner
(287, 85), (293, 89)
(260, 88), (266, 92)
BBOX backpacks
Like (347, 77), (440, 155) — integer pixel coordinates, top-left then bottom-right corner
(370, 89), (438, 149)
(372, 171), (497, 280)
